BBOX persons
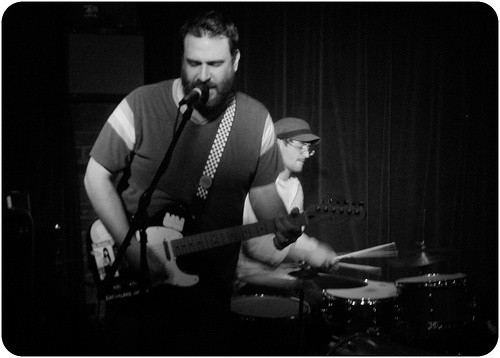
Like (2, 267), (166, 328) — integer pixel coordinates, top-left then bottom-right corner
(84, 12), (302, 354)
(237, 116), (340, 313)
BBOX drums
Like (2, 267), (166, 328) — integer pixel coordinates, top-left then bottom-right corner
(322, 278), (402, 326)
(227, 293), (310, 341)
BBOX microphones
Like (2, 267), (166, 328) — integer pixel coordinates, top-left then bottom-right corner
(178, 83), (210, 104)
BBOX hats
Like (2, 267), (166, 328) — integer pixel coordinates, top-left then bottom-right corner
(273, 117), (321, 145)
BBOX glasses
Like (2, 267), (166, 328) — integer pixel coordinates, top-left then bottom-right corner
(283, 139), (316, 157)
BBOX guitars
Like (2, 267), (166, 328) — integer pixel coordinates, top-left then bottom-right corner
(89, 194), (368, 290)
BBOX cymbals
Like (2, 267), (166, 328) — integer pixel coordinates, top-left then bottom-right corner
(395, 274), (468, 283)
(388, 249), (452, 266)
